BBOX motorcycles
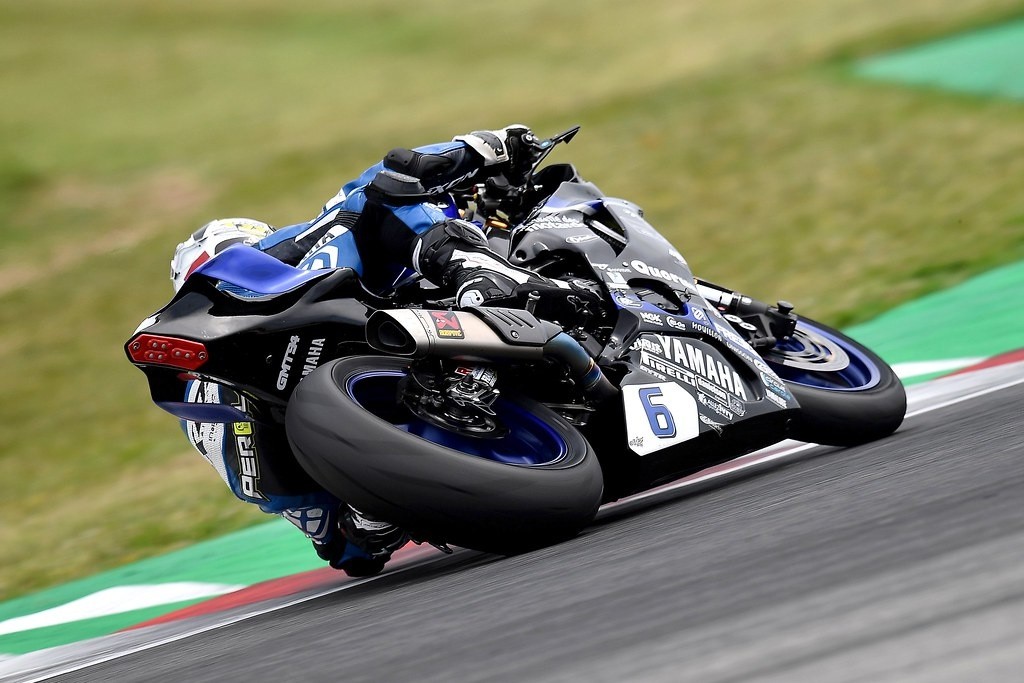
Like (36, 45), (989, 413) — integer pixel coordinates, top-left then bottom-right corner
(127, 125), (908, 555)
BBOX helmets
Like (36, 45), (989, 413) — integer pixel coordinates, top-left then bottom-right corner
(172, 215), (279, 295)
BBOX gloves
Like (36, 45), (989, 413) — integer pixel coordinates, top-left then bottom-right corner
(451, 122), (542, 187)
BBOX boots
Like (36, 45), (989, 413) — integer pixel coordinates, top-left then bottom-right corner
(413, 221), (606, 326)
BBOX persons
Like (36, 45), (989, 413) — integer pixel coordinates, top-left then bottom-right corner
(169, 124), (604, 577)
